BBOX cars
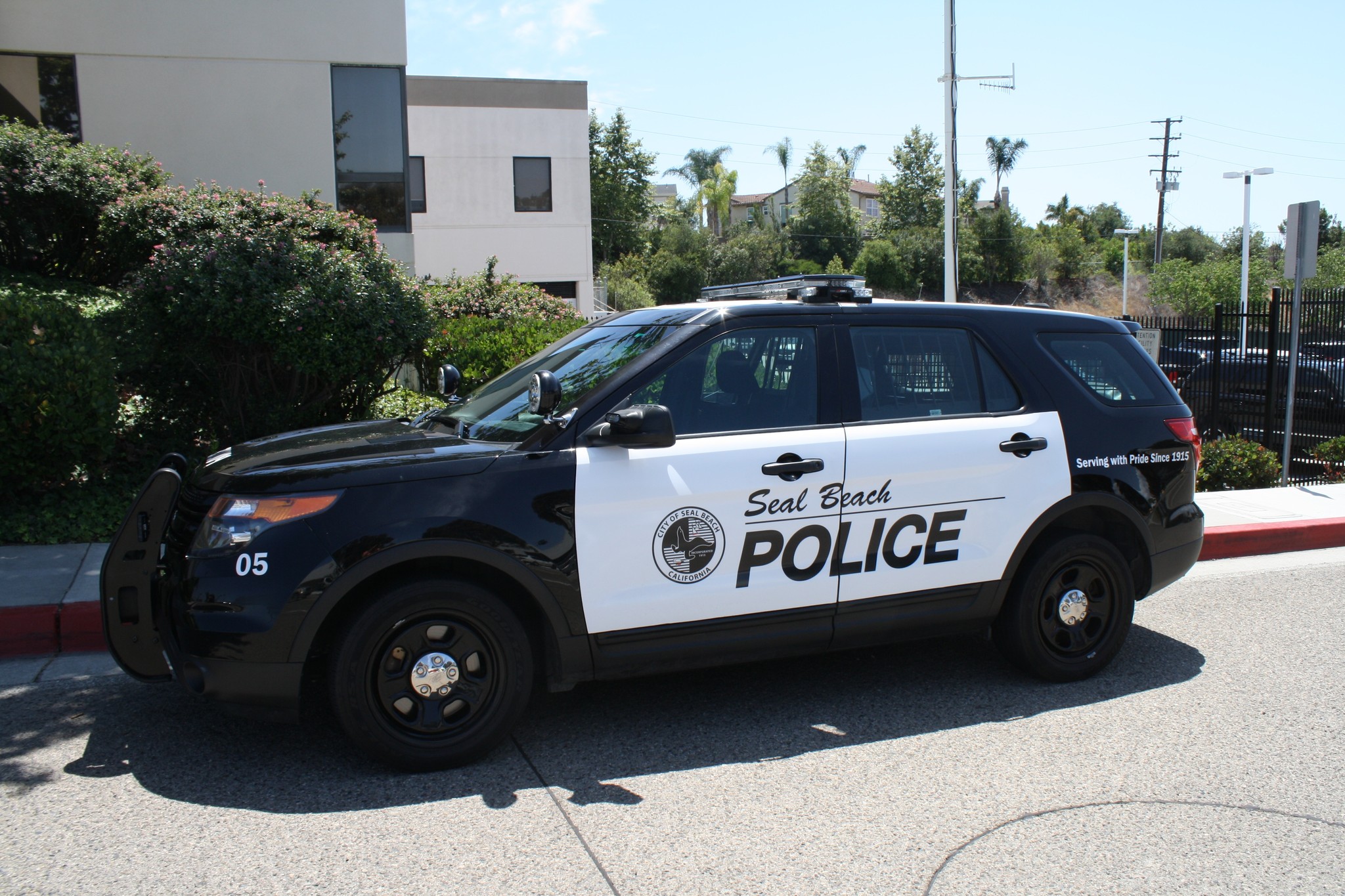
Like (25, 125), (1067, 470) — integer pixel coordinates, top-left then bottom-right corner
(1154, 330), (1345, 424)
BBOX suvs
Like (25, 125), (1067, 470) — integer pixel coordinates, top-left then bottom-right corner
(100, 277), (1204, 776)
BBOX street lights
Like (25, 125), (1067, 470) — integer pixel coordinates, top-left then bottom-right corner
(1223, 167), (1273, 361)
(1114, 229), (1140, 316)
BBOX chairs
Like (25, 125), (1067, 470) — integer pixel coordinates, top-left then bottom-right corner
(715, 344), (767, 428)
